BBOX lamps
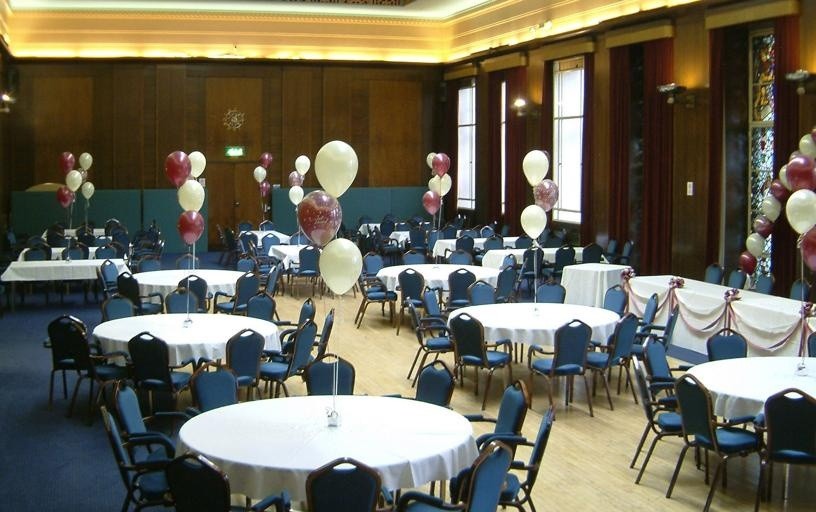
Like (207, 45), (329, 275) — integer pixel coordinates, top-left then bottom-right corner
(510, 98), (542, 120)
(0, 92), (16, 112)
(658, 82), (695, 109)
(785, 71), (816, 97)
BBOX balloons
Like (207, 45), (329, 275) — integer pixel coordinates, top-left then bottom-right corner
(57, 150), (94, 208)
(165, 150), (208, 247)
(519, 149), (557, 242)
(253, 141), (363, 296)
(422, 152), (452, 216)
(740, 125), (816, 276)
